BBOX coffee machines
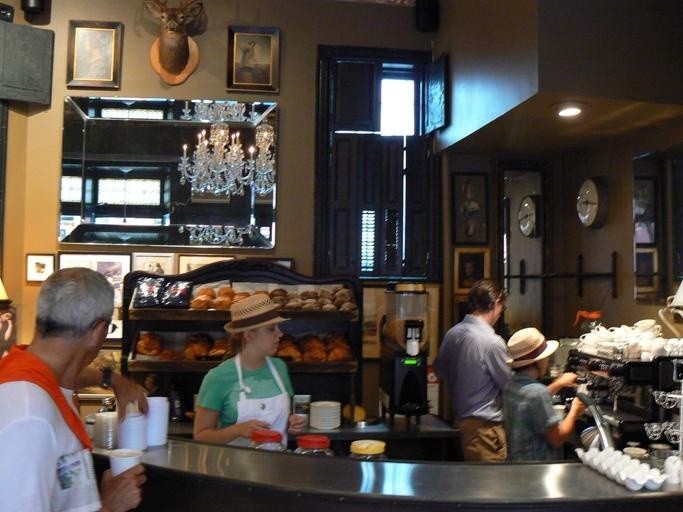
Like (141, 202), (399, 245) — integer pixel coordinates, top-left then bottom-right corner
(563, 350), (683, 436)
(371, 272), (440, 423)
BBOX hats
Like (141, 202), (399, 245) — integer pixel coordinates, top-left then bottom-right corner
(504, 327), (559, 370)
(223, 293), (292, 334)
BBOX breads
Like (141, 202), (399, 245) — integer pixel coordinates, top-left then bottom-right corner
(135, 286), (356, 362)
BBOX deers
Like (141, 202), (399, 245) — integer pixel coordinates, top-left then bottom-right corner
(143, 0), (205, 79)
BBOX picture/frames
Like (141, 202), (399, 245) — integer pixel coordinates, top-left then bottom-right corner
(447, 244), (493, 296)
(173, 253), (237, 275)
(55, 249), (132, 347)
(450, 167), (492, 247)
(634, 248), (659, 294)
(665, 142), (682, 281)
(188, 178), (232, 206)
(223, 20), (283, 96)
(418, 49), (449, 136)
(633, 174), (657, 246)
(253, 184), (273, 204)
(244, 255), (297, 271)
(23, 250), (57, 283)
(64, 15), (125, 91)
(126, 249), (176, 276)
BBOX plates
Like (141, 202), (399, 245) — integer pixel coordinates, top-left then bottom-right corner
(310, 399), (341, 430)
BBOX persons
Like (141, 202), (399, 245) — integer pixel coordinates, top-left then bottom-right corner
(193, 294), (308, 451)
(0, 308), (15, 342)
(0, 266), (148, 512)
(502, 327), (589, 464)
(433, 278), (515, 462)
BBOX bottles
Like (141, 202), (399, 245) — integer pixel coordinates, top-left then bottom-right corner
(349, 440), (388, 461)
(621, 443), (681, 485)
(247, 428), (285, 454)
(293, 434), (334, 456)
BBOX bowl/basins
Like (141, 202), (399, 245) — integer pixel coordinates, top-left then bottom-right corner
(117, 411), (148, 450)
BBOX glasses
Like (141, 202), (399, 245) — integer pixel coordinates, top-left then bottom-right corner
(108, 322), (117, 335)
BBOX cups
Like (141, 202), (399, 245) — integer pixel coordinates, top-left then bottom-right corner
(107, 446), (142, 477)
(575, 319), (683, 361)
(145, 395), (168, 445)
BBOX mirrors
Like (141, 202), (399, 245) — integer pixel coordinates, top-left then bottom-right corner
(499, 161), (547, 334)
(629, 148), (667, 300)
(51, 93), (278, 253)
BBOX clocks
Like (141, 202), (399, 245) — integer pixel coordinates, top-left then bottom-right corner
(515, 193), (539, 240)
(573, 174), (608, 230)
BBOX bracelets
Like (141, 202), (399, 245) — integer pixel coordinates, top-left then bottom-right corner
(99, 365), (112, 389)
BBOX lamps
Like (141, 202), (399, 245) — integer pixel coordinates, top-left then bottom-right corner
(171, 113), (275, 199)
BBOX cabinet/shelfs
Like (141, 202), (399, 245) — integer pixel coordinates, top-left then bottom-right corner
(121, 260), (359, 376)
